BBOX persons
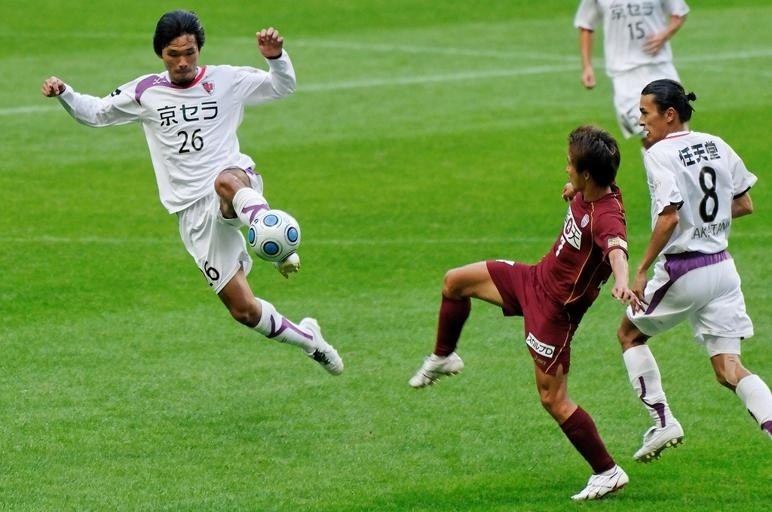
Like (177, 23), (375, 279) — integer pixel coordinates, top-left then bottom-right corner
(409, 125), (642, 500)
(42, 11), (344, 376)
(574, 1), (691, 156)
(616, 79), (772, 464)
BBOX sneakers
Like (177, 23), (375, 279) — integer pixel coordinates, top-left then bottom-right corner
(279, 253), (300, 278)
(570, 465), (630, 500)
(300, 317), (344, 377)
(408, 353), (464, 389)
(633, 417), (684, 463)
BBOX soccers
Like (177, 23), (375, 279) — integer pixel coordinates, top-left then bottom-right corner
(249, 210), (301, 261)
